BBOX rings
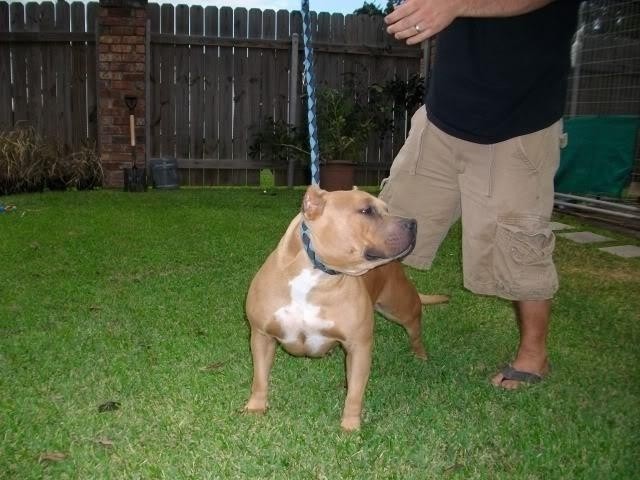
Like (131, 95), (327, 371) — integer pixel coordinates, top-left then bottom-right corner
(415, 25), (421, 33)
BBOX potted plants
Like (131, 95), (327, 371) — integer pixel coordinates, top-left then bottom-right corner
(2, 118), (102, 199)
(243, 61), (404, 192)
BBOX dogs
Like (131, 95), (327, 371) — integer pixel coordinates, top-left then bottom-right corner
(240, 181), (453, 436)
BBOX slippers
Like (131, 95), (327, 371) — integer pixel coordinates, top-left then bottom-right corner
(492, 364), (544, 390)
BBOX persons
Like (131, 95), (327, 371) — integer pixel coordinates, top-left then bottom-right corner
(376, 0), (568, 391)
